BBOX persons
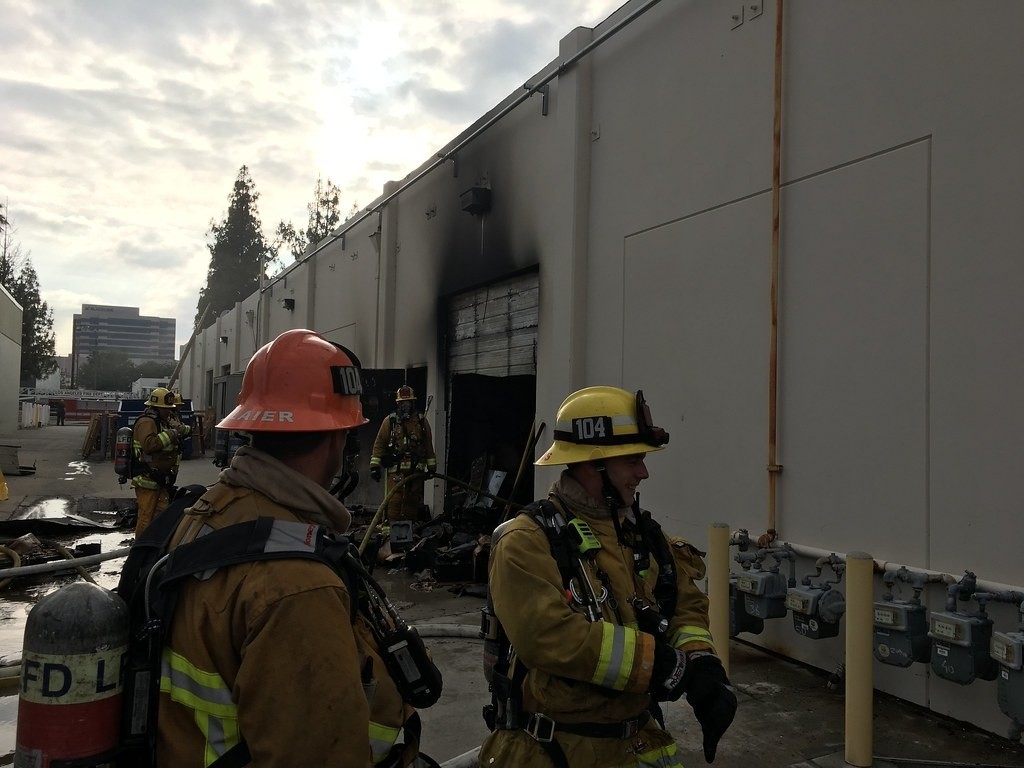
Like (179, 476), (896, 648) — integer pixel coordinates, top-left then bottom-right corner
(369, 385), (437, 530)
(114, 329), (449, 768)
(477, 386), (738, 767)
(56, 399), (65, 426)
(130, 387), (191, 541)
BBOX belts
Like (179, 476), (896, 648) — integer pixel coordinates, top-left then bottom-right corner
(520, 713), (650, 740)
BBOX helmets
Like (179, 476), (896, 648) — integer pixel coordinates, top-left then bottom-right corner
(144, 387), (176, 408)
(216, 329), (371, 433)
(395, 386), (417, 401)
(533, 386), (671, 466)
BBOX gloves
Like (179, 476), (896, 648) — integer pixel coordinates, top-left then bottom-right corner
(683, 659), (738, 763)
(651, 640), (688, 703)
(370, 467), (380, 482)
(425, 470), (434, 479)
